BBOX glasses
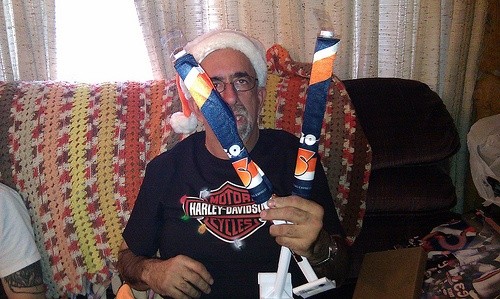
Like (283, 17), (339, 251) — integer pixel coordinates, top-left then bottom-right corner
(212, 76), (258, 93)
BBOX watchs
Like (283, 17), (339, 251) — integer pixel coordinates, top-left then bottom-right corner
(308, 234), (339, 268)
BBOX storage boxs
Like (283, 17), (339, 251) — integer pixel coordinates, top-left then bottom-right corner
(353, 246), (428, 299)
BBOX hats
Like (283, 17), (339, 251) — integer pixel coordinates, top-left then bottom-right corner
(170, 29), (268, 134)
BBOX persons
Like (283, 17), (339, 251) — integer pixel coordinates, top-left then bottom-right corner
(116, 30), (353, 299)
(0, 180), (47, 299)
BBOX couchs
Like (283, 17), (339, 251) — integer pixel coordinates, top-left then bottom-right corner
(0, 76), (470, 299)
